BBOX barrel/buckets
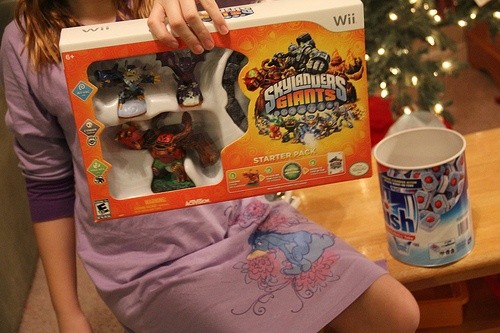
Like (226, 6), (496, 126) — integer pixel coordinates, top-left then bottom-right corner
(371, 126), (475, 269)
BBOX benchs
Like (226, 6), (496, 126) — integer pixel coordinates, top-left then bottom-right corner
(292, 127), (500, 292)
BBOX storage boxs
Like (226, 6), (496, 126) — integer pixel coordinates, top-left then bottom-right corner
(59, 0), (373, 222)
(413, 283), (470, 329)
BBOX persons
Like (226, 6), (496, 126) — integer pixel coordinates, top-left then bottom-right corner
(0, 0), (421, 333)
